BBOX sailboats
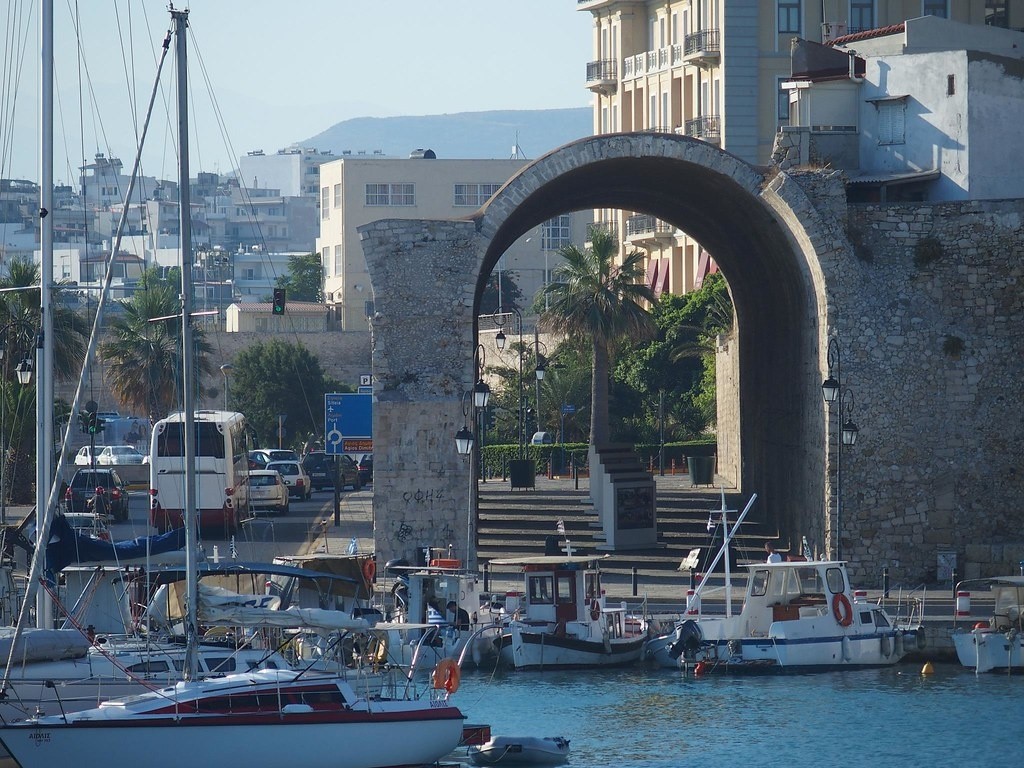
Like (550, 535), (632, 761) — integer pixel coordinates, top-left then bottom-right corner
(0, 2), (1024, 768)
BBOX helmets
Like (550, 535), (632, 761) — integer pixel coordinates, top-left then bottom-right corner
(96, 486), (104, 495)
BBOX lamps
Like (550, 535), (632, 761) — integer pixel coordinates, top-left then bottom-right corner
(338, 292), (342, 299)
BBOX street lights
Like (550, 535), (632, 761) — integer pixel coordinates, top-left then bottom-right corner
(523, 326), (550, 432)
(492, 304), (523, 460)
(454, 344), (492, 575)
(821, 337), (859, 567)
(220, 364), (234, 412)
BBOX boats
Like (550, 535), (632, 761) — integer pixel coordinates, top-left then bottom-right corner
(640, 483), (926, 677)
(951, 563), (1024, 678)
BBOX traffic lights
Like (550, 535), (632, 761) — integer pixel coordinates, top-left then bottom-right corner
(272, 288), (285, 314)
(78, 415), (85, 433)
(524, 408), (535, 423)
(88, 412), (96, 435)
(515, 409), (520, 421)
(95, 418), (106, 435)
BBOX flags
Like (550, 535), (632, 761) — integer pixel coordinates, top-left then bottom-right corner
(803, 540), (812, 562)
(230, 538), (240, 559)
(348, 537), (357, 555)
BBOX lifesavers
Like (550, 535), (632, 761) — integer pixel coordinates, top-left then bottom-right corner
(591, 600), (600, 620)
(433, 658), (460, 693)
(833, 592), (852, 627)
(356, 559), (376, 580)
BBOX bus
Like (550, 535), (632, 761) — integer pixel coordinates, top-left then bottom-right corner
(97, 411), (122, 419)
(147, 410), (258, 535)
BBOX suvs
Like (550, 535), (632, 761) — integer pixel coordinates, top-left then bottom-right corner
(248, 451), (276, 470)
(264, 460), (312, 502)
(357, 453), (373, 487)
(65, 468), (132, 521)
(301, 450), (362, 491)
(253, 449), (299, 461)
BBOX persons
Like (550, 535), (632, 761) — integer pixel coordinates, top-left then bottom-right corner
(89, 486), (110, 514)
(765, 542), (781, 574)
(447, 601), (469, 631)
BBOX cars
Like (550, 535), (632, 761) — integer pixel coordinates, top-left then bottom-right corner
(249, 470), (291, 516)
(96, 446), (145, 465)
(63, 513), (109, 543)
(74, 446), (107, 466)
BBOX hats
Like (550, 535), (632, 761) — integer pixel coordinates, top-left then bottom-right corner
(446, 601), (456, 609)
